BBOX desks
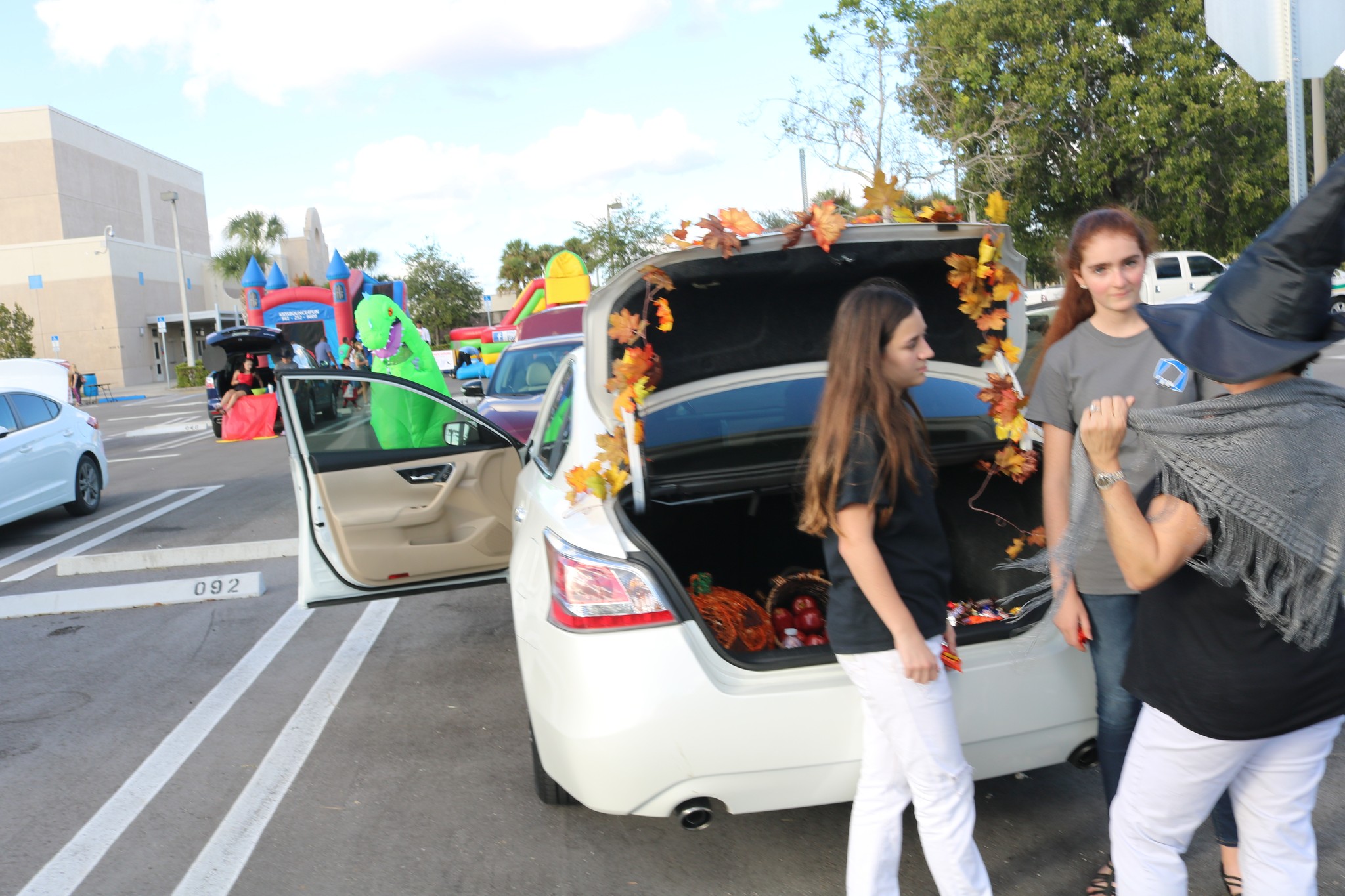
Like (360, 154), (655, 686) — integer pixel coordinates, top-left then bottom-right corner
(86, 383), (118, 405)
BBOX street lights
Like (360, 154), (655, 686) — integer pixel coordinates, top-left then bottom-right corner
(160, 190), (196, 380)
(607, 202), (623, 276)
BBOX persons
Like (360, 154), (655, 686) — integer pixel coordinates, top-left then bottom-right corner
(452, 346), (482, 379)
(415, 320), (431, 348)
(796, 287), (994, 896)
(272, 344), (302, 436)
(313, 334), (371, 410)
(1077, 244), (1344, 896)
(213, 354), (264, 414)
(1024, 208), (1243, 896)
(70, 364), (83, 407)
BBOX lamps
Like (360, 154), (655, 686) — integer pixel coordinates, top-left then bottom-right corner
(95, 224), (114, 254)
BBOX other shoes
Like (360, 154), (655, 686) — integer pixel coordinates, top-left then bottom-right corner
(215, 406), (226, 416)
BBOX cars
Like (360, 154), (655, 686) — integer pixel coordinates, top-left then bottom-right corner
(461, 302), (587, 445)
(0, 358), (110, 527)
(268, 221), (1099, 834)
(204, 325), (337, 439)
(1024, 251), (1344, 336)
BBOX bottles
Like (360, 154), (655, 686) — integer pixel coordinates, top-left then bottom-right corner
(268, 382), (274, 393)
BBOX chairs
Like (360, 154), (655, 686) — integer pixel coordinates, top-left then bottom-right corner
(516, 363), (556, 392)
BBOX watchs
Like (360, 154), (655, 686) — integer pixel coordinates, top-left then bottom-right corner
(1094, 468), (1126, 491)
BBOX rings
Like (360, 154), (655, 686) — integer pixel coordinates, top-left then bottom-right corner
(1090, 404), (1101, 412)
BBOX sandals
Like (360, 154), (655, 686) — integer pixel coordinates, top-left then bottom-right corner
(1218, 861), (1242, 896)
(1085, 854), (1117, 896)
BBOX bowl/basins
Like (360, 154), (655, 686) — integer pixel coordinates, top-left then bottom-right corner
(251, 388), (266, 395)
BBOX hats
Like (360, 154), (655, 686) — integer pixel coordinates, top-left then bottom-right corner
(276, 346), (297, 357)
(1135, 150), (1345, 384)
